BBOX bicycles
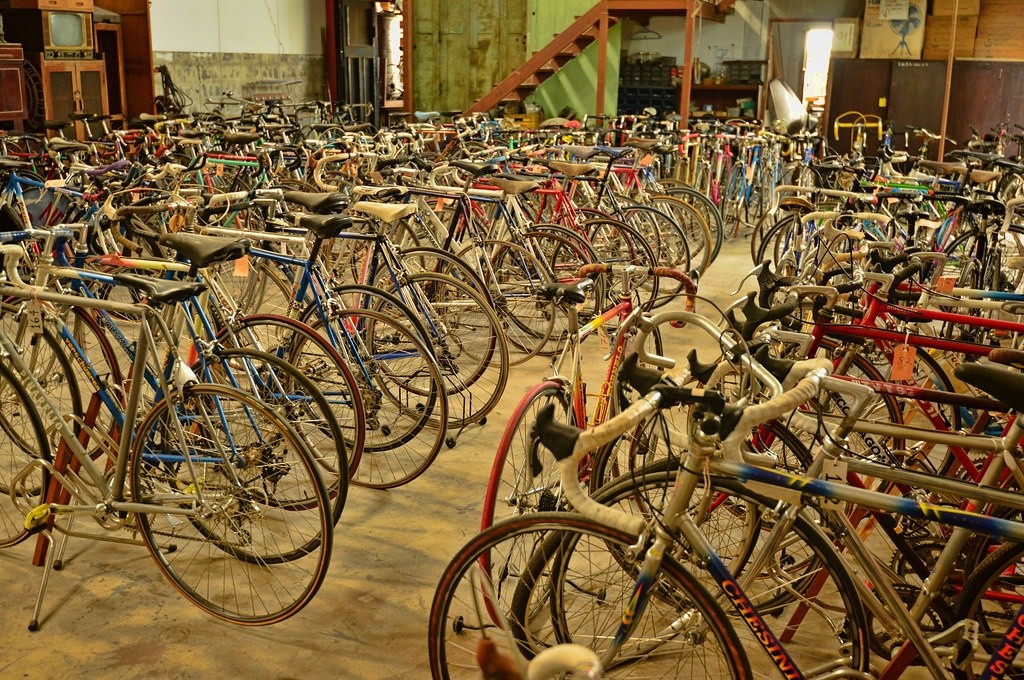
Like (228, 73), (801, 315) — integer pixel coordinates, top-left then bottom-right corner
(0, 113), (1024, 680)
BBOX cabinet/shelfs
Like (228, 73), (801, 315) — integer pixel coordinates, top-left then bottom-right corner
(94, 23), (129, 130)
(28, 52), (112, 143)
(616, 83), (763, 123)
(820, 58), (1024, 170)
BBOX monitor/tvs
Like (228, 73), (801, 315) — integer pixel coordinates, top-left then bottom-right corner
(40, 10), (94, 60)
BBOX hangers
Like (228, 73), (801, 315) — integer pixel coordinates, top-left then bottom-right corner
(628, 26), (663, 40)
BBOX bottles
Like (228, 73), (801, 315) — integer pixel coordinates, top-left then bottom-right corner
(927, 347), (972, 394)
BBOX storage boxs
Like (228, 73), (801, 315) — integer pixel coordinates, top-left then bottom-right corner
(504, 112), (544, 131)
(829, 0), (1024, 62)
